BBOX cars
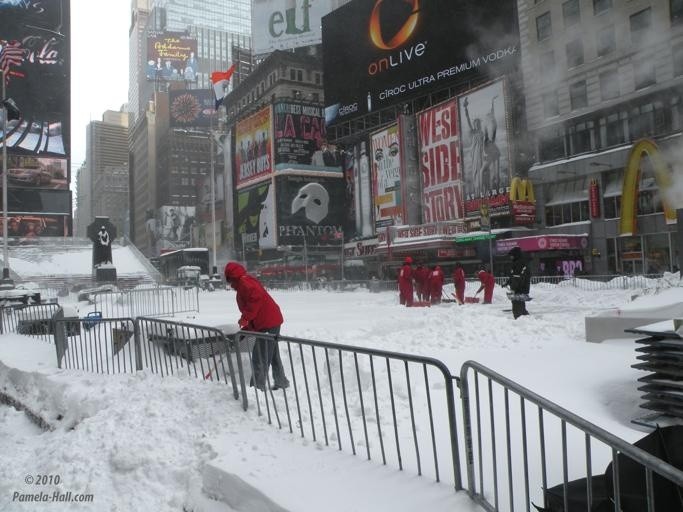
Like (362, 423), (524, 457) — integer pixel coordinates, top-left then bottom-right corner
(7, 164), (51, 185)
(175, 269), (222, 287)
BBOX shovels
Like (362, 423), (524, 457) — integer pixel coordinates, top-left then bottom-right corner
(442, 289), (456, 303)
(465, 292), (480, 303)
(413, 293), (431, 307)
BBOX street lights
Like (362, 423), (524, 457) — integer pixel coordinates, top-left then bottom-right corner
(0, 98), (20, 290)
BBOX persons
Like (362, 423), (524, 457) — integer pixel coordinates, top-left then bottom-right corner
(474, 270), (495, 304)
(224, 261), (290, 392)
(463, 95), (501, 192)
(501, 247), (533, 320)
(170, 208), (179, 241)
(398, 256), (465, 307)
(147, 51), (198, 82)
(97, 225), (111, 266)
(240, 132), (269, 162)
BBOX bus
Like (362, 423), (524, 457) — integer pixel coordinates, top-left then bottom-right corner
(161, 252), (209, 276)
